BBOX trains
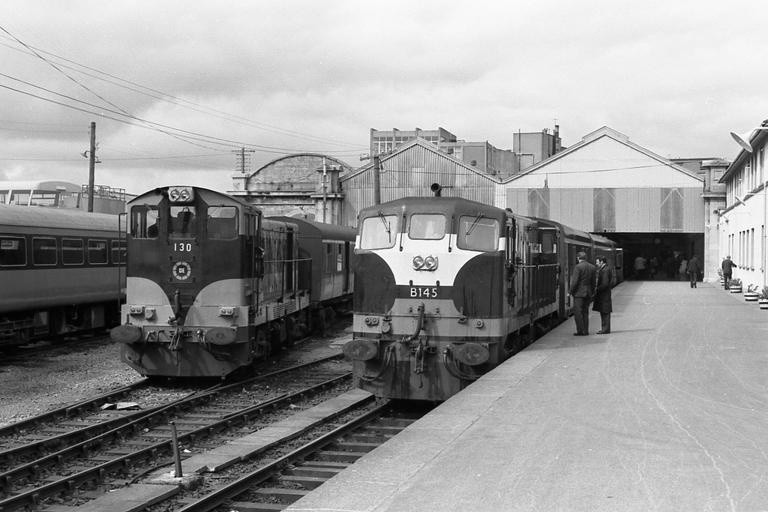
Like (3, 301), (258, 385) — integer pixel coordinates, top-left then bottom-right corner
(121, 183), (363, 389)
(331, 180), (627, 403)
(0, 204), (129, 353)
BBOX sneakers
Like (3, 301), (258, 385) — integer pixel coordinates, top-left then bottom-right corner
(574, 332), (588, 336)
(598, 331), (610, 334)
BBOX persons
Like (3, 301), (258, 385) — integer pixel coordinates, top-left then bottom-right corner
(633, 248), (701, 289)
(567, 251), (598, 336)
(590, 254), (613, 335)
(721, 256), (736, 290)
